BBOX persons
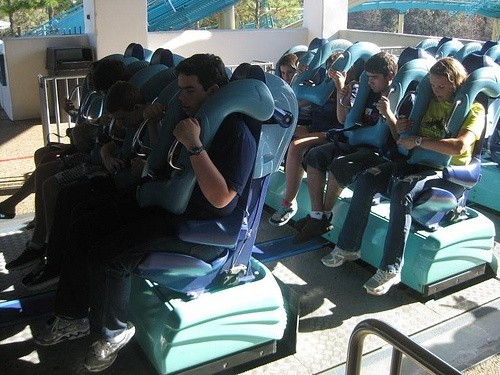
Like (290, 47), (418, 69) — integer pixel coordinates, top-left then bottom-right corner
(320, 56), (485, 295)
(280, 54), (315, 129)
(22, 81), (147, 291)
(293, 51), (418, 245)
(0, 60), (126, 230)
(34, 54), (258, 372)
(270, 49), (363, 226)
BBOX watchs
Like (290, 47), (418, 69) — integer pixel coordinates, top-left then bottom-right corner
(189, 145), (206, 156)
(415, 136), (424, 147)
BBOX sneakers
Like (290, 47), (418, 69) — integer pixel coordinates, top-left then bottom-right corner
(4, 245), (46, 272)
(22, 262), (61, 291)
(293, 211), (334, 244)
(268, 199), (298, 226)
(83, 320), (136, 372)
(361, 268), (402, 296)
(320, 244), (361, 268)
(34, 315), (90, 346)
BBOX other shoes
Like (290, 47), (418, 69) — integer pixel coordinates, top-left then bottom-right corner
(0, 202), (15, 219)
(25, 217), (36, 231)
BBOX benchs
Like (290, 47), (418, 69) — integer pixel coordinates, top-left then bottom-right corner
(32, 34), (500, 375)
